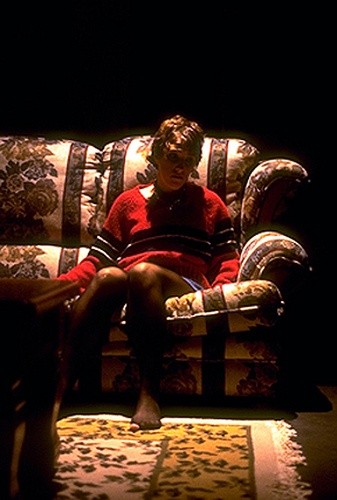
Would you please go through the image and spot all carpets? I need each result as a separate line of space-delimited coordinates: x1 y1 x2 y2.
52 412 313 500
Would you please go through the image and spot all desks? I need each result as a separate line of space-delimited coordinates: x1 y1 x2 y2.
0 278 83 499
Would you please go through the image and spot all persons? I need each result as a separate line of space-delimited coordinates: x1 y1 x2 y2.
47 112 241 430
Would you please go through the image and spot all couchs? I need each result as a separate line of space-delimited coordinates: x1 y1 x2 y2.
0 135 312 400
